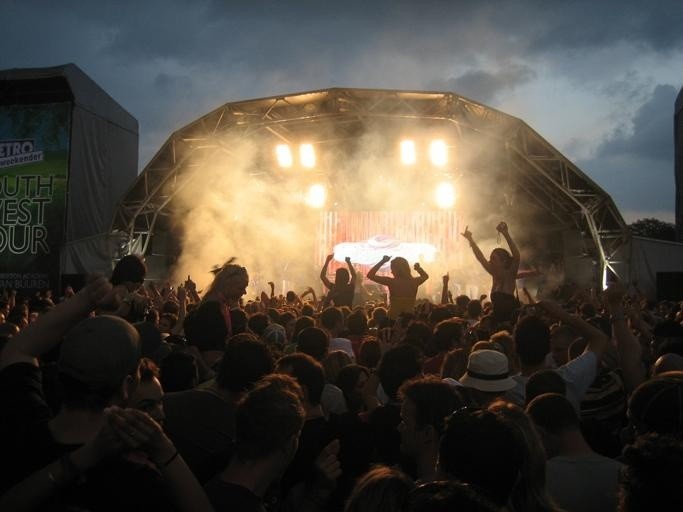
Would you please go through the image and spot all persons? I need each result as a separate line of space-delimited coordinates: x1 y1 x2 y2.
0 220 682 511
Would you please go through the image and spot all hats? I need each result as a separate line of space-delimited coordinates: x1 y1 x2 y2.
459 349 517 393
